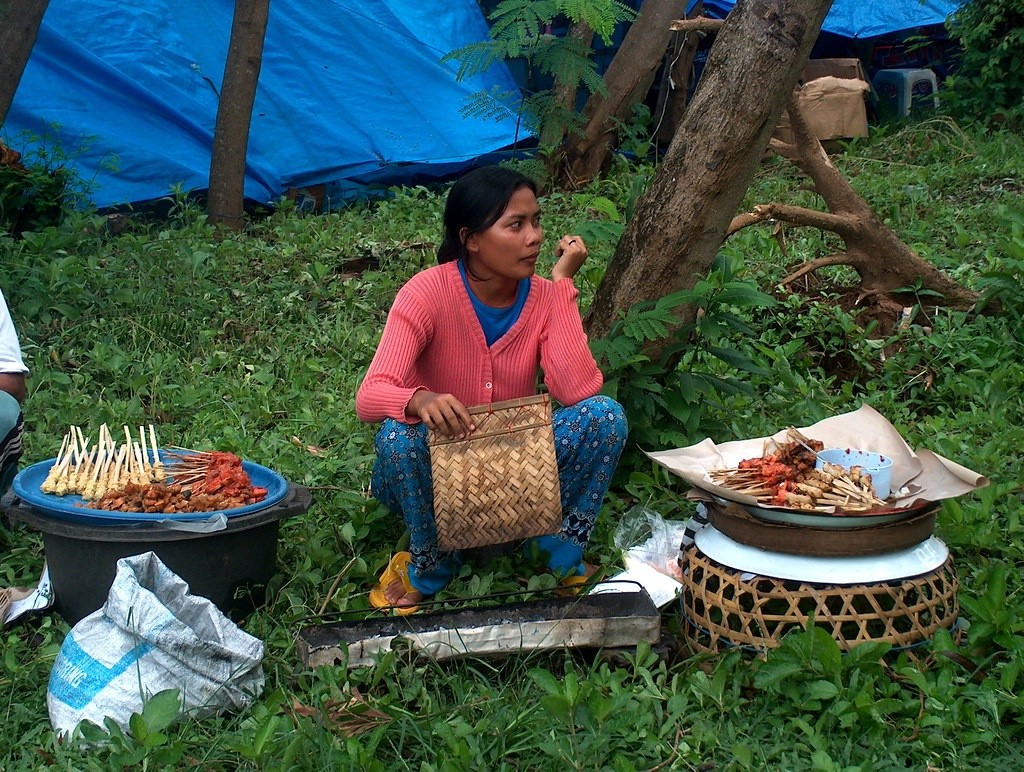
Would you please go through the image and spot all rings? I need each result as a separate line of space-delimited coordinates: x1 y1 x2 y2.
568 240 576 245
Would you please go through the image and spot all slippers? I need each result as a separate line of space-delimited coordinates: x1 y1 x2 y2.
369 551 422 616
518 562 607 596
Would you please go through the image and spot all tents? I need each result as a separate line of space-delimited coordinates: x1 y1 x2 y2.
0 0 977 216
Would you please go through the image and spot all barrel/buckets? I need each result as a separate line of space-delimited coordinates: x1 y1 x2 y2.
6 484 312 629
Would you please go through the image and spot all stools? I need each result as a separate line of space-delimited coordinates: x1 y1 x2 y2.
868 68 939 122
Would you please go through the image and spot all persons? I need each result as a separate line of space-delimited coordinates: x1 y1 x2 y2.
356 167 627 617
0 286 30 492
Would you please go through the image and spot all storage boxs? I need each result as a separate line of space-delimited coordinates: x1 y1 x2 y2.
773 58 879 155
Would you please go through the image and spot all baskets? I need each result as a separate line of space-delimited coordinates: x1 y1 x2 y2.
679 543 961 677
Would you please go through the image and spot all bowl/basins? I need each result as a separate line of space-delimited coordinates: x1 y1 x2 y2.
816 449 893 500
